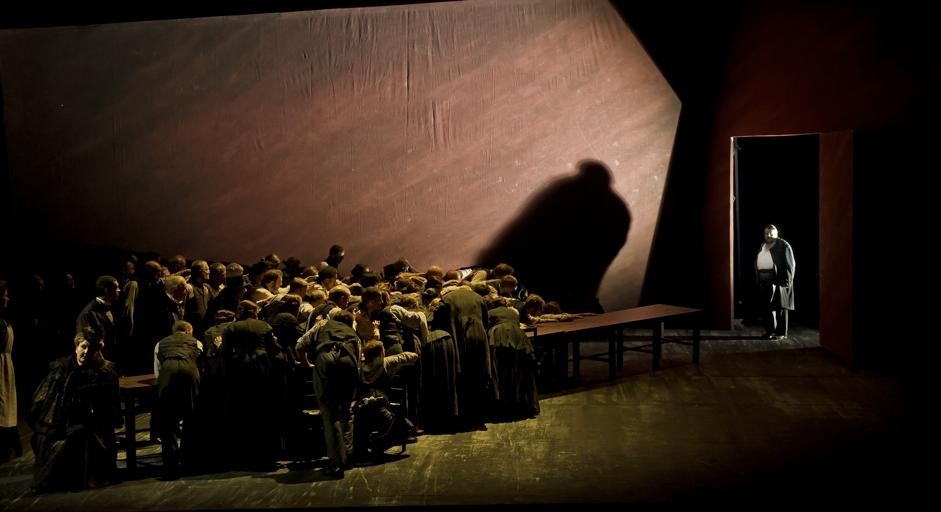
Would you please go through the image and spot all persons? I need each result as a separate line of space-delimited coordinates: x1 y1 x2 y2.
1 243 595 478
756 223 798 343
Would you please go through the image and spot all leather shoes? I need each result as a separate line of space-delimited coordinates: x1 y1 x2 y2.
763 332 787 339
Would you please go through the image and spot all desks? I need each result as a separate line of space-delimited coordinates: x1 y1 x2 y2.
118 303 705 480
